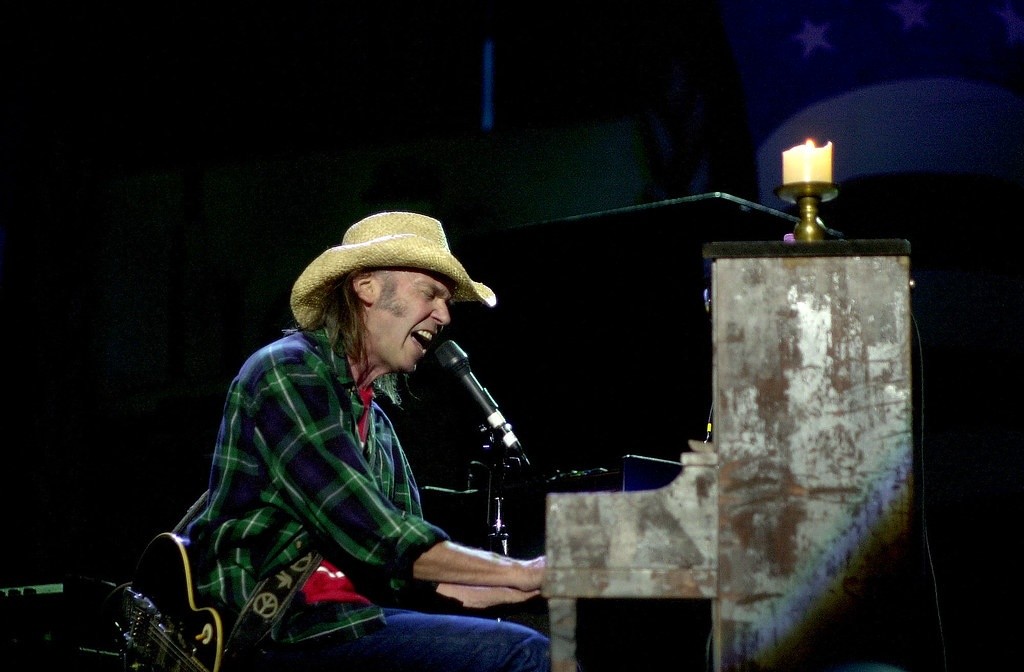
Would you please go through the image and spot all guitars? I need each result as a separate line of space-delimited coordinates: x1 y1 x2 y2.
104 527 234 672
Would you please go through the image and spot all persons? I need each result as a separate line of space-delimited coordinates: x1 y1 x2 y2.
179 212 581 672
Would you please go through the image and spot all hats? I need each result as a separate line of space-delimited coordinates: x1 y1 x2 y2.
289 211 497 331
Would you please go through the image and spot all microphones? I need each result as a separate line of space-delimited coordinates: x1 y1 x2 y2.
435 340 531 467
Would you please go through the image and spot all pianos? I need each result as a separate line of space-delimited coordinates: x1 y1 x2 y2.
544 235 926 672
